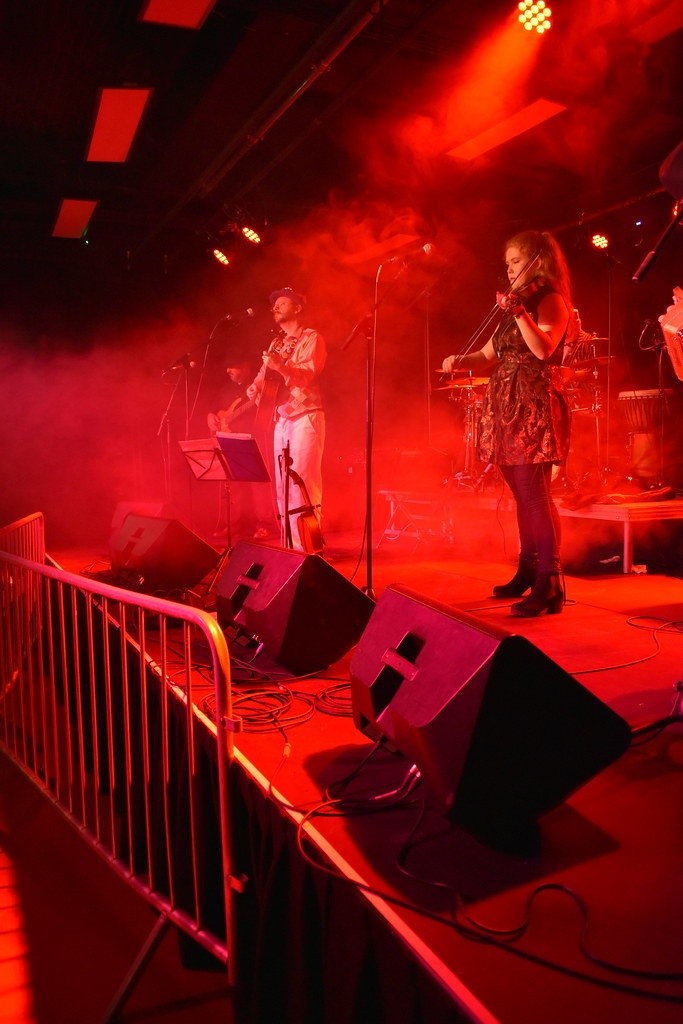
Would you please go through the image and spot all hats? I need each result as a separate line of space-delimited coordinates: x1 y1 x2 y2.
268 287 307 309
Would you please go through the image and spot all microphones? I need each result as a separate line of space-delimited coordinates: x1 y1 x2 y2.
172 361 196 370
226 308 254 320
380 243 435 266
602 252 620 264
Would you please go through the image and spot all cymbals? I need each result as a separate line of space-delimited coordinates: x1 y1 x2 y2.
446 377 490 385
435 385 478 389
585 337 608 344
435 367 468 373
567 389 581 394
574 356 616 368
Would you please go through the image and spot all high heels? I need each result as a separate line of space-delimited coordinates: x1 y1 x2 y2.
511 556 564 615
494 553 538 598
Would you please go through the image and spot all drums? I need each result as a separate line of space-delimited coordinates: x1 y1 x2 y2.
466 401 483 417
618 388 672 477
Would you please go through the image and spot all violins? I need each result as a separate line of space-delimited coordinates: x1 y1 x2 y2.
502 273 548 315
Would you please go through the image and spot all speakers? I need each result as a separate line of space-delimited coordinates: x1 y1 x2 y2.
349 583 631 836
214 540 376 675
110 500 220 597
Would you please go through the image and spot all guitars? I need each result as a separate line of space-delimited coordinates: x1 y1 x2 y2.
210 397 255 439
254 330 288 431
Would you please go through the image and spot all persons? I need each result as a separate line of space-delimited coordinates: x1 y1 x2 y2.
245 287 328 563
206 343 290 541
550 305 596 486
442 232 574 613
